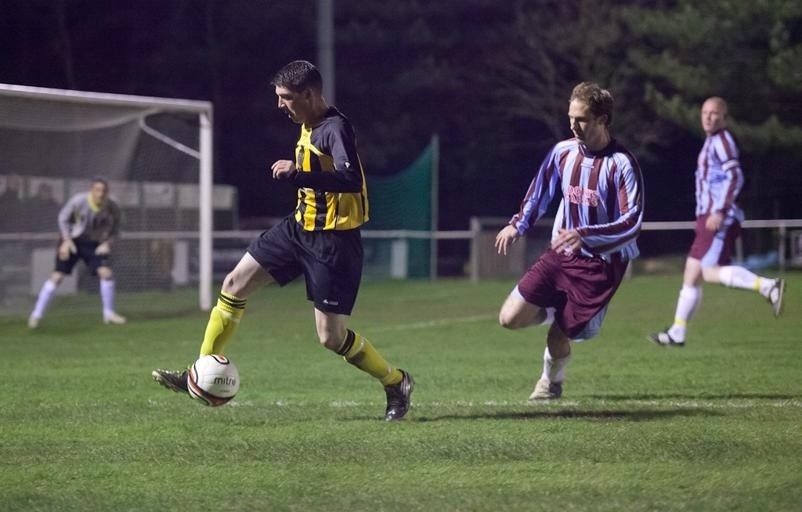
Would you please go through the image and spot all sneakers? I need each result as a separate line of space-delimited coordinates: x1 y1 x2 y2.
528 373 562 401
766 278 786 318
384 368 416 422
28 309 40 330
152 365 190 395
644 327 686 347
103 313 127 324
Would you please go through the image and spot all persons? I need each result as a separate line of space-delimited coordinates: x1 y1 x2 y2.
27 178 126 330
495 81 645 402
648 96 786 347
153 59 415 422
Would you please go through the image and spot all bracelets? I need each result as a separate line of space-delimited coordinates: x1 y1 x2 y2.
288 169 298 183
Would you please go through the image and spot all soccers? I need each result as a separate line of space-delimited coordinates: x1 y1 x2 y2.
187 354 239 408
